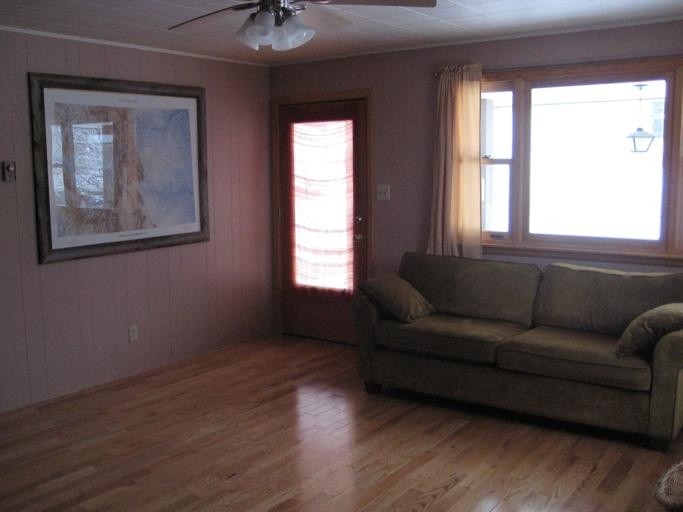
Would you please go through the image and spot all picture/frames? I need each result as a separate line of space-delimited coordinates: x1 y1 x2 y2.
27 71 209 264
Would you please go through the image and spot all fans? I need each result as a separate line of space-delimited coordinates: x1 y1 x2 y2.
167 0 437 31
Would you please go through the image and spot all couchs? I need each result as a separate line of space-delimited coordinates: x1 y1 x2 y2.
352 251 683 453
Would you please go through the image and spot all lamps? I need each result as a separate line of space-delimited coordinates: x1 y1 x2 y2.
626 126 655 152
233 11 315 52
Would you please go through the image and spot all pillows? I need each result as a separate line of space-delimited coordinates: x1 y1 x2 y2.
618 304 683 355
358 272 436 324
